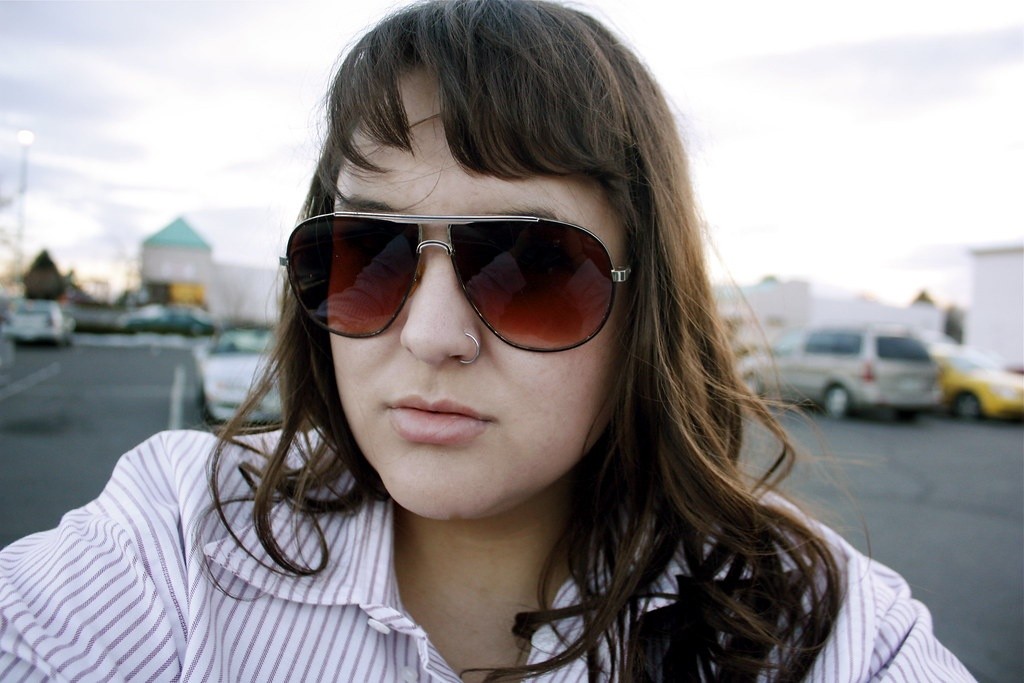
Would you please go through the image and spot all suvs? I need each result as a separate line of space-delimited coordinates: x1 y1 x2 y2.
760 320 944 423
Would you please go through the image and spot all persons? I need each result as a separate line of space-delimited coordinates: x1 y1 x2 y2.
0 1 976 683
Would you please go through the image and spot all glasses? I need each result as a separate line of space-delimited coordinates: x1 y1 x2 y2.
281 210 629 354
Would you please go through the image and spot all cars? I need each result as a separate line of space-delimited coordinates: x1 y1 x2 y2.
902 337 1024 428
10 296 77 345
112 300 211 336
196 328 288 428
